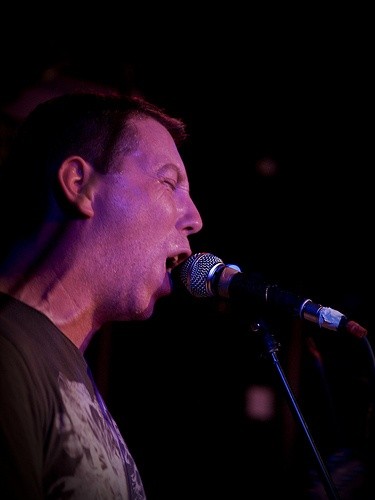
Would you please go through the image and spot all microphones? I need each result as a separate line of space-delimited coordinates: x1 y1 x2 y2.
180 251 367 339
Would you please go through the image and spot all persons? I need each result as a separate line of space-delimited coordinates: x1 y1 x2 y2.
0 83 202 500
